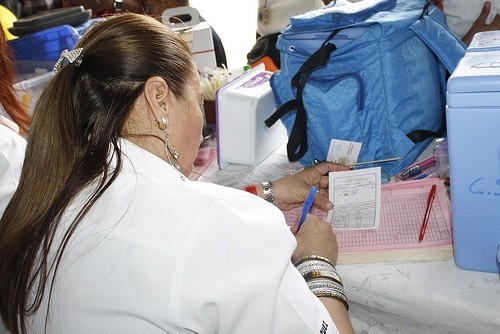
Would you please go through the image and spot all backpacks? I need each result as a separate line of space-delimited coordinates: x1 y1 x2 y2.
276 0 465 176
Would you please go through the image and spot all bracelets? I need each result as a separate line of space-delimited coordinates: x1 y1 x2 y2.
260 180 279 209
242 184 258 197
290 256 351 310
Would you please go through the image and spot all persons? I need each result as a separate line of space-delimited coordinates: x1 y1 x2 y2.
0 14 354 334
0 19 31 222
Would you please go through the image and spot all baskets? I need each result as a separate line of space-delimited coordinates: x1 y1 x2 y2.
203 100 216 136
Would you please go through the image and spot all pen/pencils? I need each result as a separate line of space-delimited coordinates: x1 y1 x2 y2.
294 185 319 236
401 154 436 180
419 184 437 241
